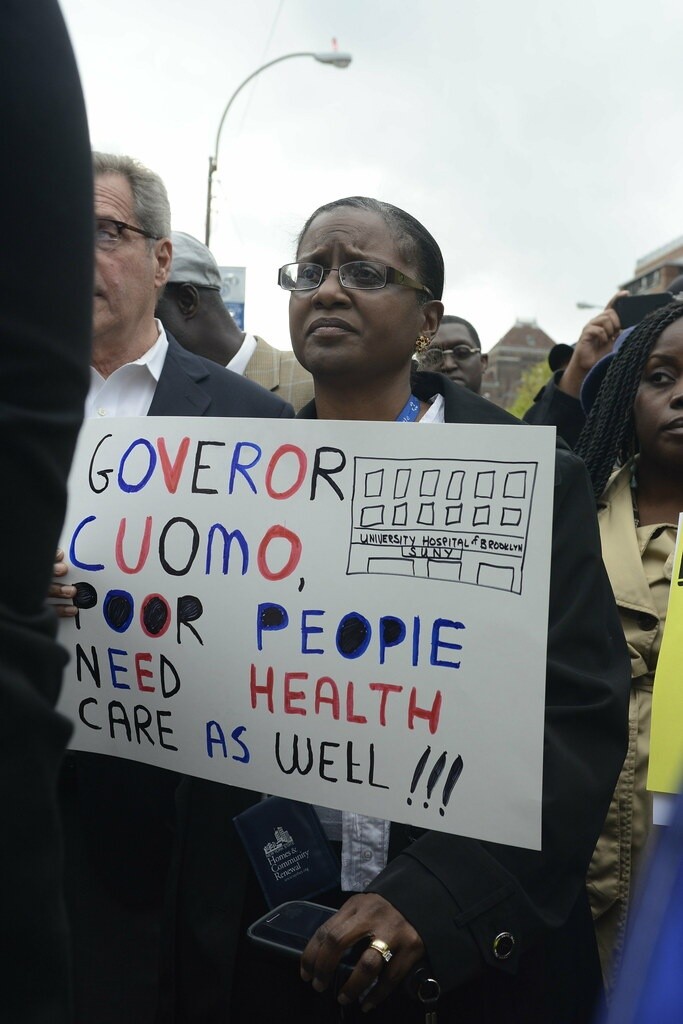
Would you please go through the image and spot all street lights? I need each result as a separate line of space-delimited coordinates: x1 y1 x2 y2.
201 50 357 250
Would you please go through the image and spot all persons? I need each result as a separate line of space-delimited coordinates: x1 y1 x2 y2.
0 0 682 1024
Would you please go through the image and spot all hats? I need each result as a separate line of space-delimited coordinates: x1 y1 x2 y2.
167 231 223 288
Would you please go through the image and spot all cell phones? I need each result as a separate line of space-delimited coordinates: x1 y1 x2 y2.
246 901 373 973
614 292 673 330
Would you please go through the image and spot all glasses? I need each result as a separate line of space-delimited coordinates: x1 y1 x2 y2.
419 345 481 368
95 217 162 254
279 260 433 297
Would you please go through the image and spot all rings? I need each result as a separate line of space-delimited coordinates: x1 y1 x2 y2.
368 937 394 964
